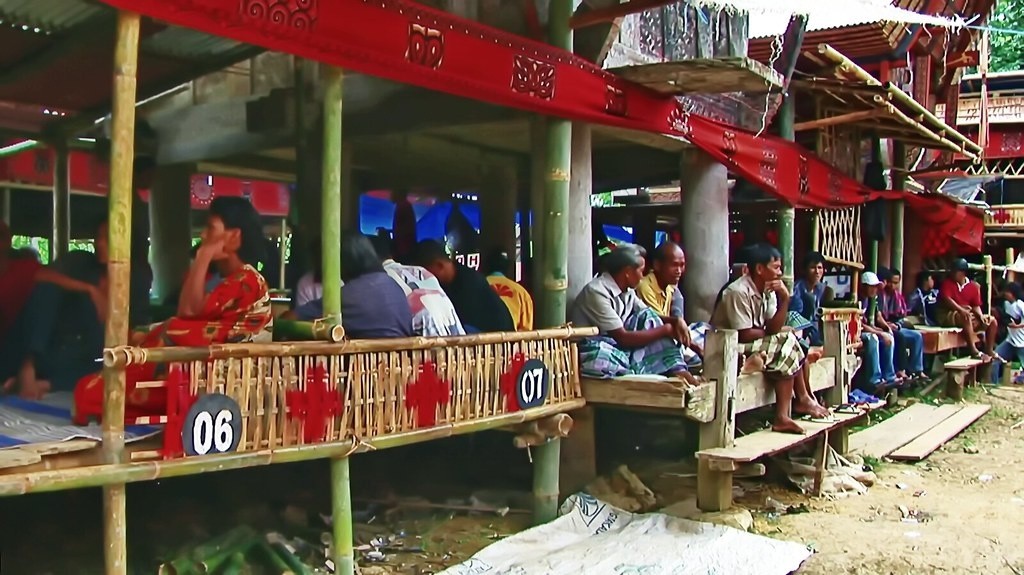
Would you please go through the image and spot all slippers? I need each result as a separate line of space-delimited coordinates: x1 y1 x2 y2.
833 401 871 414
990 352 999 359
801 414 841 423
874 375 933 388
971 351 985 360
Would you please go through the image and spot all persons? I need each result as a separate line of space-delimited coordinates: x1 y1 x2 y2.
0 155 1023 443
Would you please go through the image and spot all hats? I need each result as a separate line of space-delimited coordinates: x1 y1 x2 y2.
861 271 884 286
